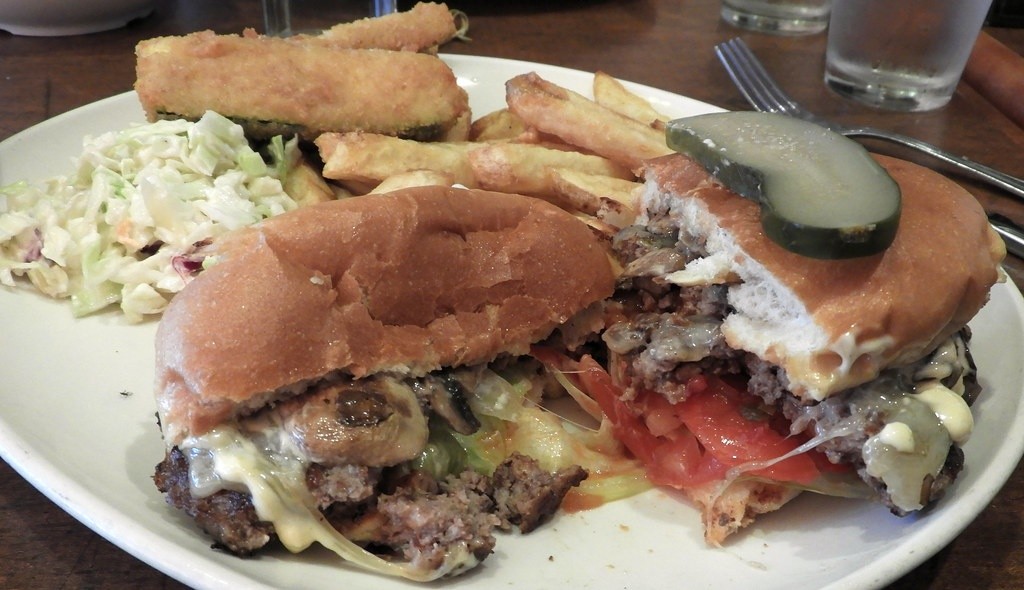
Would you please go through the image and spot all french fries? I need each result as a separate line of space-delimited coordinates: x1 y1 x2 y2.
312 69 676 236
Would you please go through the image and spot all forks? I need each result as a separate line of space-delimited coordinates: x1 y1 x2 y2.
711 35 1024 206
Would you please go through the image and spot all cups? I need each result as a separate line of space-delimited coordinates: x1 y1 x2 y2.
822 0 995 113
719 0 831 37
258 1 399 42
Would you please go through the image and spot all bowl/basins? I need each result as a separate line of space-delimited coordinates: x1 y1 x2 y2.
0 0 153 37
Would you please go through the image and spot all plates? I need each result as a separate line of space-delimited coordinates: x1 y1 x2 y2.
0 53 1024 590
